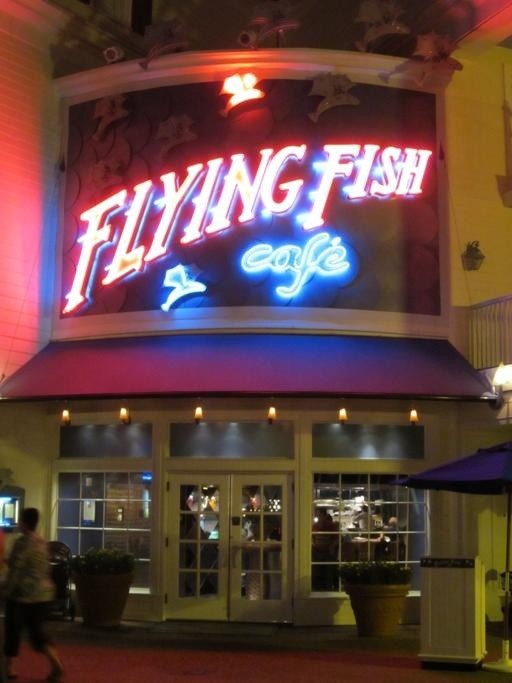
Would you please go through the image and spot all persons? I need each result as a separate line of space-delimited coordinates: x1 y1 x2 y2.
313 500 405 590
0 506 66 682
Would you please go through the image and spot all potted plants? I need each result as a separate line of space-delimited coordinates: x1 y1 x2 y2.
336 559 417 637
66 545 136 628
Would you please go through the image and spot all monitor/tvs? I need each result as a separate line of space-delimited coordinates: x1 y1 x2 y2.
0 497 19 525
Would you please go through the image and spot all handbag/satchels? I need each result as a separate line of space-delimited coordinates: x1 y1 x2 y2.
52 563 68 598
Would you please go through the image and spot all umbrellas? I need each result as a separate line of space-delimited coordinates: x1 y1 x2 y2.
392 439 512 661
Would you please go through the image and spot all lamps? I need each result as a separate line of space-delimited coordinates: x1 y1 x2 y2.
194 407 204 425
463 240 485 271
409 408 419 425
266 407 277 426
61 410 73 428
102 44 126 64
338 408 348 426
120 407 131 425
236 29 257 49
